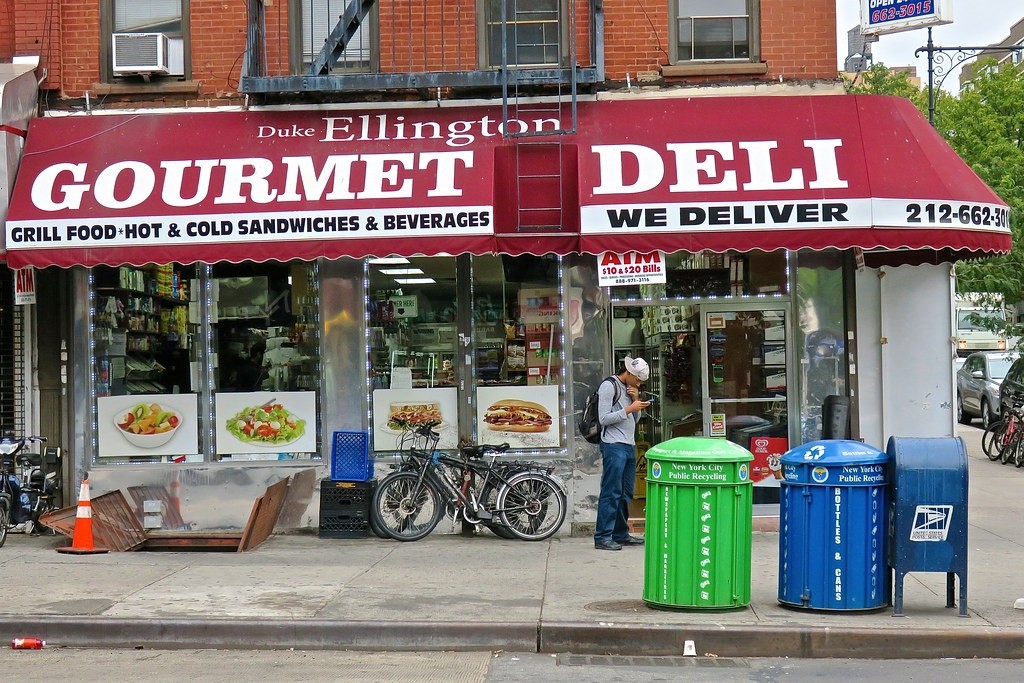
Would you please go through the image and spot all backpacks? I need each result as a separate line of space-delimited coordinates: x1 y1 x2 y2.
579 377 622 444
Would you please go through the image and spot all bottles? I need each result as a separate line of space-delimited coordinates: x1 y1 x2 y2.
296 375 314 386
384 333 397 348
371 370 390 389
378 300 394 320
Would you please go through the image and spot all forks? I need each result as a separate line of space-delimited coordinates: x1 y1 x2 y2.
243 398 276 421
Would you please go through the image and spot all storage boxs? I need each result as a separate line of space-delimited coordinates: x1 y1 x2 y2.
319 429 379 539
640 304 689 345
517 288 560 386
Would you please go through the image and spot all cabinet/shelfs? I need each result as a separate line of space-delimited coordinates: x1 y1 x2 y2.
761 311 787 396
94 286 189 393
219 316 319 391
370 326 408 389
504 320 526 382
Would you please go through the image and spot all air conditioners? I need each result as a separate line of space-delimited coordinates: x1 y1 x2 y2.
112 32 170 75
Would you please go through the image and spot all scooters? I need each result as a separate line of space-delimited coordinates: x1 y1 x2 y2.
0 430 65 548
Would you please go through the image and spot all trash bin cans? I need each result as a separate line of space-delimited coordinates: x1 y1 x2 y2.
726 415 768 436
777 439 889 614
890 435 969 619
643 436 755 613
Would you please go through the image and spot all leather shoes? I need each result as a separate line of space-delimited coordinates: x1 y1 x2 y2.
595 539 622 550
615 535 645 546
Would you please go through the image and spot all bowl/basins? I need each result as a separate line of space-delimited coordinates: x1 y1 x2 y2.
114 404 183 448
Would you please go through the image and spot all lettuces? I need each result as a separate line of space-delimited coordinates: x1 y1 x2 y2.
226 406 305 444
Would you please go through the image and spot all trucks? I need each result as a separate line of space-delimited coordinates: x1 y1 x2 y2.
954 306 1007 359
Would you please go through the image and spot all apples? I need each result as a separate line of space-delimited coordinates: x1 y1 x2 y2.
117 413 134 428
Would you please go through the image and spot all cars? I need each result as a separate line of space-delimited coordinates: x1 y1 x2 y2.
998 354 1024 426
956 349 1022 431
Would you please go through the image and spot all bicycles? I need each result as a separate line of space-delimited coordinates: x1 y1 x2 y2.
982 392 1024 470
367 415 567 542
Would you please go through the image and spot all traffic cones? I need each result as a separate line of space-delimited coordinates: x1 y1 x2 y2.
54 479 111 556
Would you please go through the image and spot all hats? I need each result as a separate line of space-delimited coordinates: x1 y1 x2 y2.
625 356 650 381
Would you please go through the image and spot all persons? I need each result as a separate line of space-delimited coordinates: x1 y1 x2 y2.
594 356 651 550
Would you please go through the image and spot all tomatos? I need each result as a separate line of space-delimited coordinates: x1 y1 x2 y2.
243 404 293 437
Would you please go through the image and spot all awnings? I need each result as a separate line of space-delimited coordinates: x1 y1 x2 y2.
3 95 1012 271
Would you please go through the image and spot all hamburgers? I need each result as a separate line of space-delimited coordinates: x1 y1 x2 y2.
387 402 442 430
482 400 552 432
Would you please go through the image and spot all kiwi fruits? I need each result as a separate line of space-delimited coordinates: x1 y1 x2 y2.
132 404 149 421
154 412 169 426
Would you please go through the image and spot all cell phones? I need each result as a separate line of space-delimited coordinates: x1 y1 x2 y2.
648 399 654 402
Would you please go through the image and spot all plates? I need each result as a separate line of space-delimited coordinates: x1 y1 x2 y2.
381 420 450 436
228 408 304 446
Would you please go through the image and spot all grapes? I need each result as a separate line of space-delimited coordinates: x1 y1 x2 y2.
169 416 180 428
